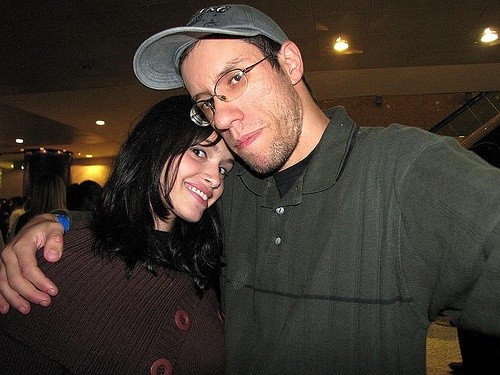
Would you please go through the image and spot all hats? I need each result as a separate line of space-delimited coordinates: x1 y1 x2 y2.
133 4 290 90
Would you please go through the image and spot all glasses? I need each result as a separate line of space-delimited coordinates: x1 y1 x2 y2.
190 53 272 127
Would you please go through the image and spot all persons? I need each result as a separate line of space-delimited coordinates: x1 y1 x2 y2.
0 93 235 375
0 4 500 375
0 172 102 252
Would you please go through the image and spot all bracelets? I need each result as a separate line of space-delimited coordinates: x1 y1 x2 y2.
56 213 70 233
